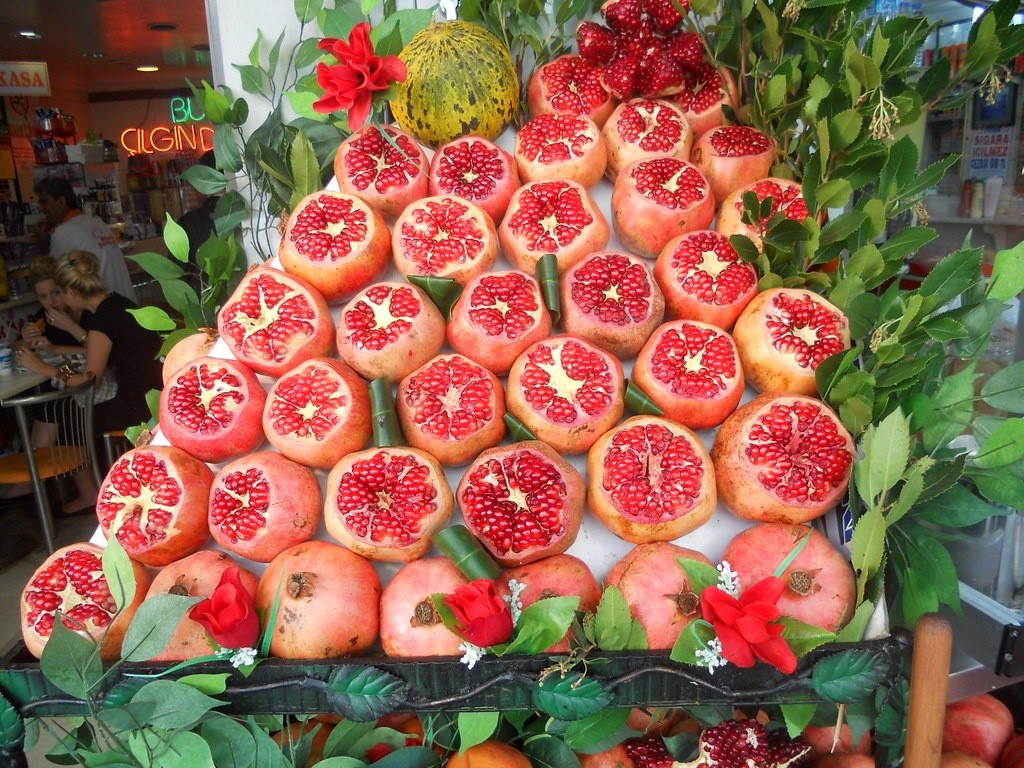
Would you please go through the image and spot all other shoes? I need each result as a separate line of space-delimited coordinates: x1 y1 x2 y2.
50 500 98 518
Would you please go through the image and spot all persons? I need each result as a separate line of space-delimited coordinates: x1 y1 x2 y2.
33 175 138 303
168 149 226 304
15 251 163 518
12 255 93 395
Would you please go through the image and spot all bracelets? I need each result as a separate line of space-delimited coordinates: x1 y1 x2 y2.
81 333 88 345
51 365 72 391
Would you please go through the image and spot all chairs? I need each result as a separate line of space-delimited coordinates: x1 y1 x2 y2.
0 371 103 559
102 356 167 469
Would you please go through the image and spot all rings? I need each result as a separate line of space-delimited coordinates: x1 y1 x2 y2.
50 318 54 322
16 349 21 354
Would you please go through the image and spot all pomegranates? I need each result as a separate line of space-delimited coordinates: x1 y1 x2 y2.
21 0 1023 768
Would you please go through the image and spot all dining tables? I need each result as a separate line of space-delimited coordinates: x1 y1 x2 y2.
0 352 74 400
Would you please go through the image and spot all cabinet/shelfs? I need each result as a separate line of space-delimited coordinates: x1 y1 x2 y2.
899 216 1024 294
0 160 204 341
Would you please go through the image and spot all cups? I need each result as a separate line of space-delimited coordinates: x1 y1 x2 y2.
0 338 11 348
0 349 13 375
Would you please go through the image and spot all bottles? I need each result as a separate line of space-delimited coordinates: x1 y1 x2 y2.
8 277 20 301
29 197 37 215
